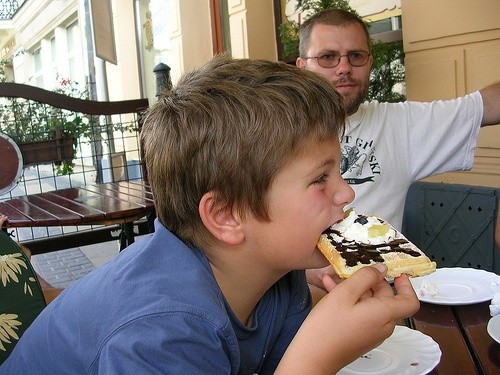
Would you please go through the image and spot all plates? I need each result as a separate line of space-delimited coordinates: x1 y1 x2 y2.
409 267 500 306
335 325 443 375
486 314 500 344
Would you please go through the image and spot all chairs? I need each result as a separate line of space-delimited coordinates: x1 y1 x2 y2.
0 215 47 366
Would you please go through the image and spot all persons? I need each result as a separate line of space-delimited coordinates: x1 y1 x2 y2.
0 46 420 375
295 8 500 234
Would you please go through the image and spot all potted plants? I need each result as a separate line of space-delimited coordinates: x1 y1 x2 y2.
0 60 140 177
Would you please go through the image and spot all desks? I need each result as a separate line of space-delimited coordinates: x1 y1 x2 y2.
0 180 156 253
393 298 500 375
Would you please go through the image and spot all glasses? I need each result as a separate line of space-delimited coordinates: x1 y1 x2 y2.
305 51 371 68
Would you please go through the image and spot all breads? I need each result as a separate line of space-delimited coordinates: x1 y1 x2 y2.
316 212 437 279
